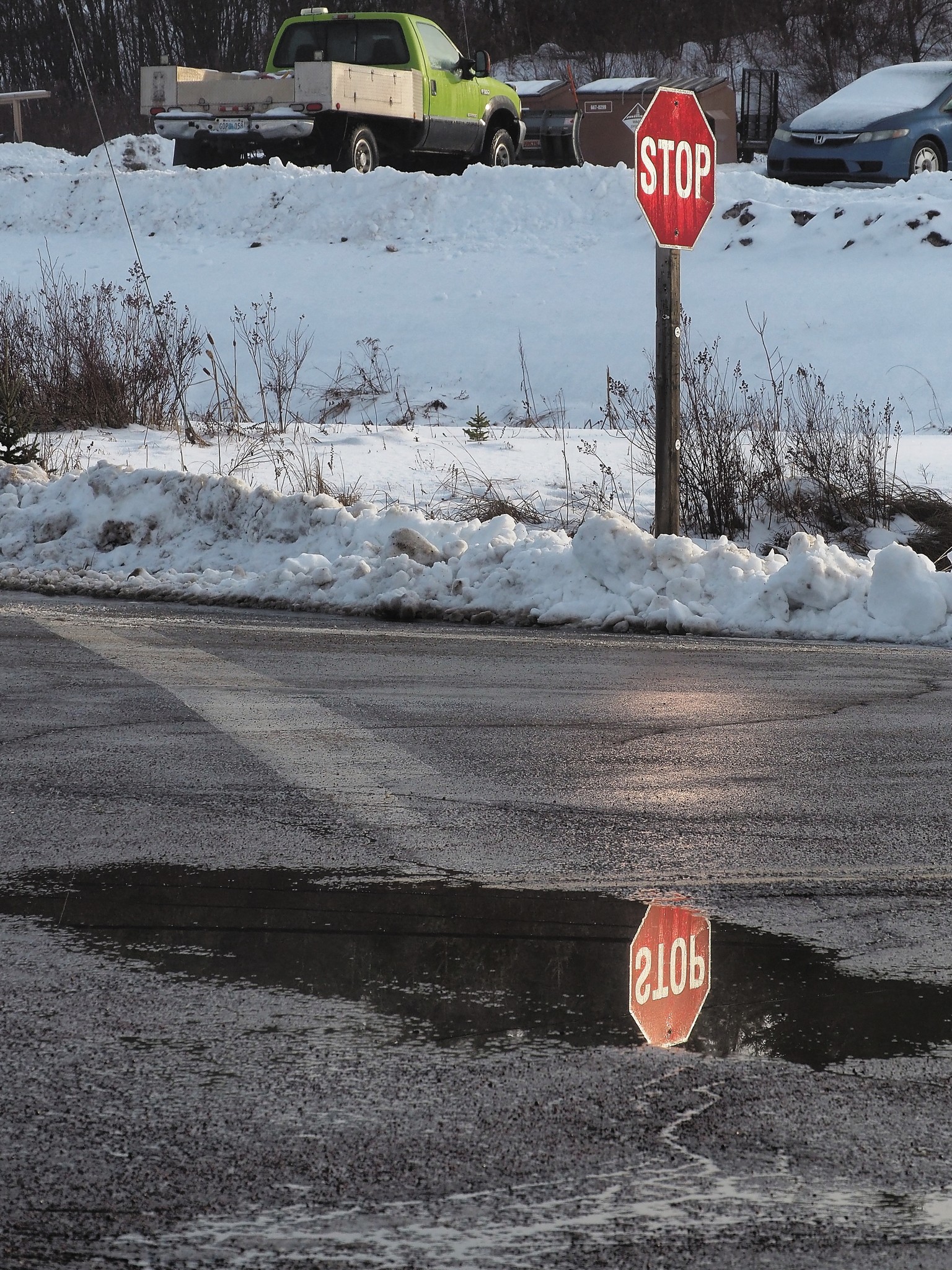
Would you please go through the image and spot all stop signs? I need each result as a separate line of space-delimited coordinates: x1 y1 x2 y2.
635 84 716 249
627 894 714 1047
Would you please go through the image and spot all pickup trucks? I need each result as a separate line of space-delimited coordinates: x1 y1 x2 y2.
139 5 528 173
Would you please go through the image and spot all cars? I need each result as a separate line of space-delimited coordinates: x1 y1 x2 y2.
767 60 952 184
